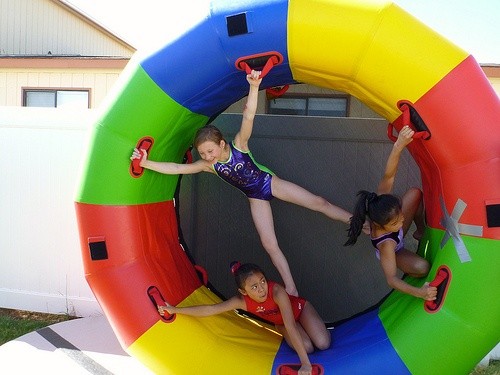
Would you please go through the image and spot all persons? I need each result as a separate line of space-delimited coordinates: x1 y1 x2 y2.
130 69 373 297
156 263 334 375
342 125 438 302
